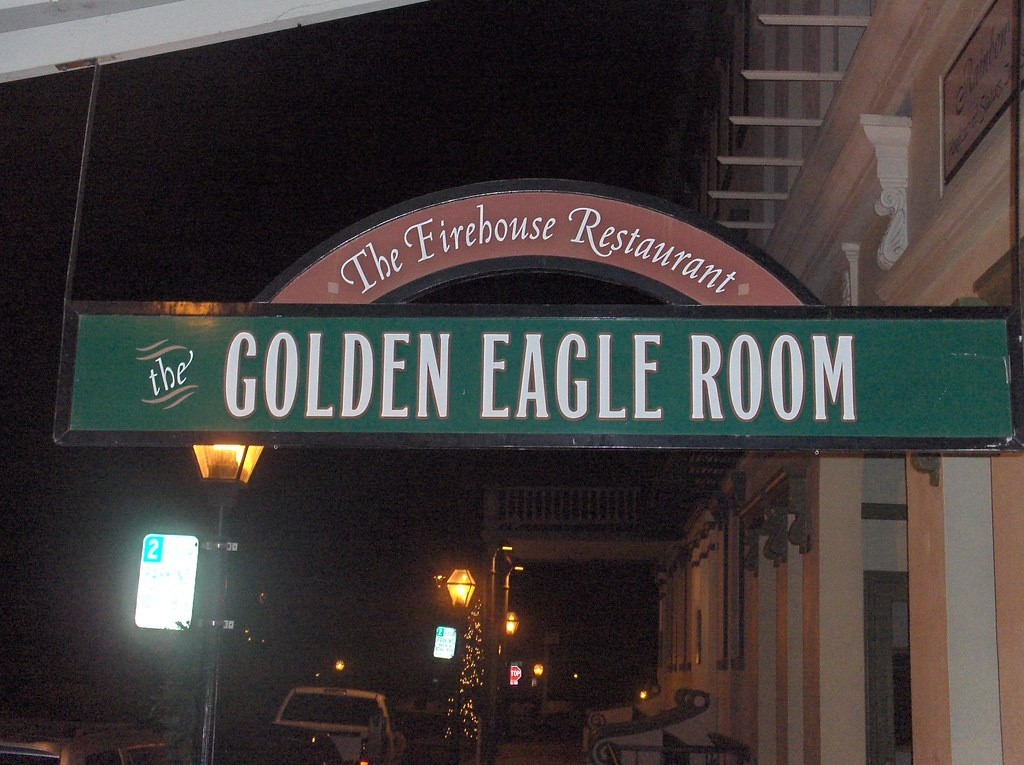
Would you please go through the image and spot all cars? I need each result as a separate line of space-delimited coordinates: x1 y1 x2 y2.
0 715 194 765
270 683 398 765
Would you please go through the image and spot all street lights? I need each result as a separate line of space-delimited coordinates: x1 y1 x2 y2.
445 567 477 765
189 444 267 765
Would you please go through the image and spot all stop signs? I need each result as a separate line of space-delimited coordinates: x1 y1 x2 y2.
511 665 522 681
